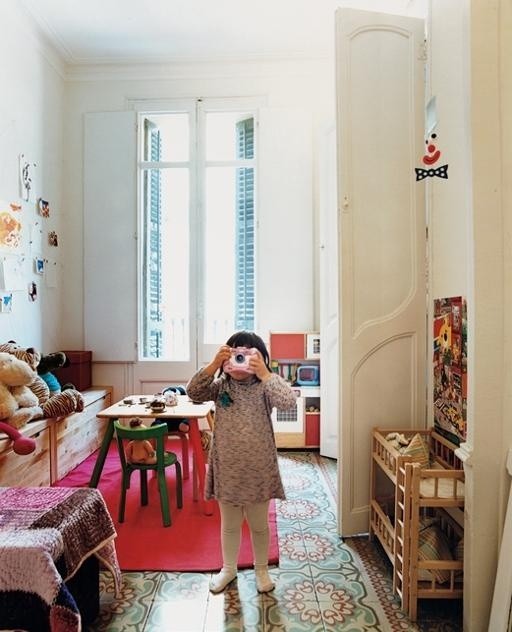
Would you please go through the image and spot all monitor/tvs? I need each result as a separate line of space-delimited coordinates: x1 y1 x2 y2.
297 366 320 386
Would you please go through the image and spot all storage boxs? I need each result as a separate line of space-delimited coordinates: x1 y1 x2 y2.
54 351 92 391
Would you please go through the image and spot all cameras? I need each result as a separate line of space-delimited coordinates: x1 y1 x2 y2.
223 347 258 375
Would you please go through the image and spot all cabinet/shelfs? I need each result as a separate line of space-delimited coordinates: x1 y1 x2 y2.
270 330 320 449
368 426 465 622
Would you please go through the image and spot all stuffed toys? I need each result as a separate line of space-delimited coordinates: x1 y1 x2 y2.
123 417 158 466
149 384 189 433
0 339 83 455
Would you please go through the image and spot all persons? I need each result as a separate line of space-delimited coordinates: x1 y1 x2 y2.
186 330 299 593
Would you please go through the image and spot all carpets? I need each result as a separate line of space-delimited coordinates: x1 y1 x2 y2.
52 439 279 572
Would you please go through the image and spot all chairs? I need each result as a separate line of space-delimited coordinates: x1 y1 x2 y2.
114 422 182 527
152 386 190 481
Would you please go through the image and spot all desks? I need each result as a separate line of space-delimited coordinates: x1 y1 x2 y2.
89 394 214 516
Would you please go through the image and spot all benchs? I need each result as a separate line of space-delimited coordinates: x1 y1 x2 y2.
0 386 112 487
